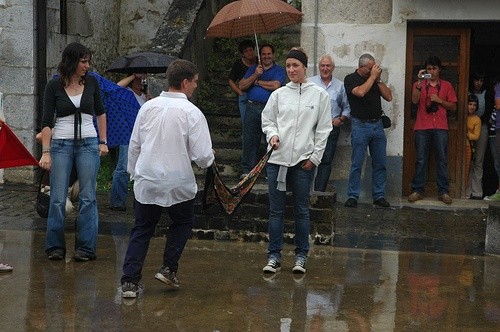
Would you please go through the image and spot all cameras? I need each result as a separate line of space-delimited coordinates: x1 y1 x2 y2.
420 72 432 80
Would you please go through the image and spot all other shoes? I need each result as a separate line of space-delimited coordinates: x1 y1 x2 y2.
0 262 13 272
408 191 424 201
473 196 480 200
107 206 127 214
372 197 390 208
48 251 64 261
345 198 357 207
73 252 97 261
313 195 332 209
438 193 453 204
484 189 500 202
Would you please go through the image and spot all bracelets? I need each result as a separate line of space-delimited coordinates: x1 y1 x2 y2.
416 85 422 90
338 117 344 122
256 79 259 86
98 141 107 144
42 148 51 152
375 79 383 83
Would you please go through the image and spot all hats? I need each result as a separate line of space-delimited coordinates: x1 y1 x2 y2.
238 39 255 54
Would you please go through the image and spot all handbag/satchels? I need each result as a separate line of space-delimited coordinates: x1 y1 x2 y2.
381 115 392 129
37 165 50 219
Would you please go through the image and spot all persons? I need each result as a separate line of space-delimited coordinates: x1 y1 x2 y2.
34 40 153 261
120 59 216 299
464 72 500 202
261 47 334 273
344 54 392 207
0 115 14 273
305 54 352 192
229 41 286 180
408 56 457 204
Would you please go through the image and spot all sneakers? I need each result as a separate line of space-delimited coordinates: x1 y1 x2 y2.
292 254 307 273
154 265 180 288
263 255 282 273
120 280 145 298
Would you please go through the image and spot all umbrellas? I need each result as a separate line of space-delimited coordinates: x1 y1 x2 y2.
52 71 141 148
204 0 304 65
105 51 181 73
